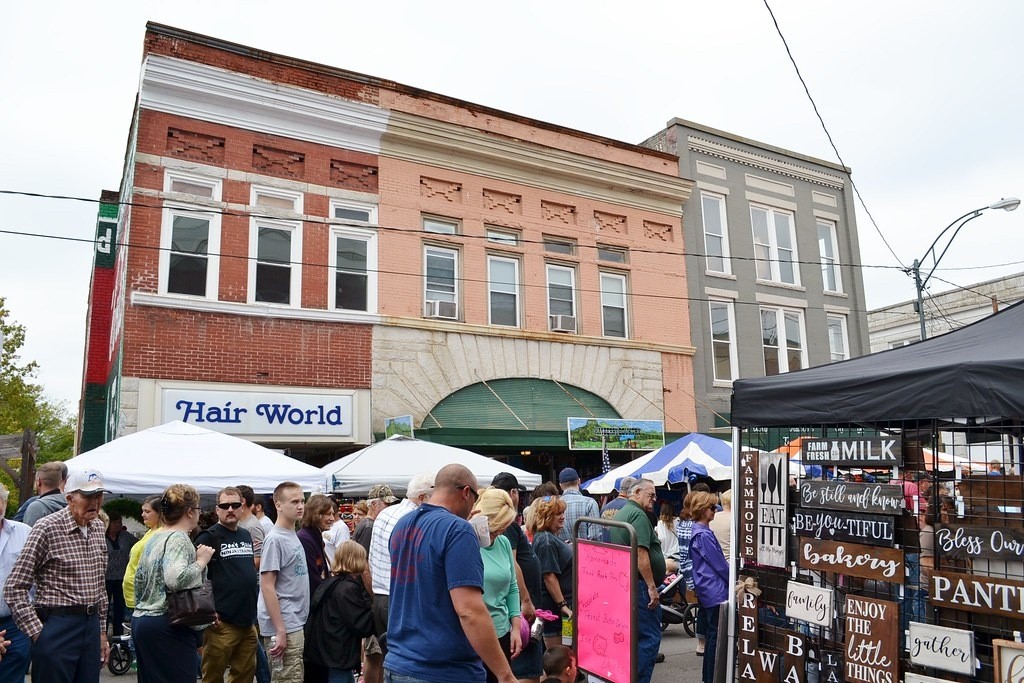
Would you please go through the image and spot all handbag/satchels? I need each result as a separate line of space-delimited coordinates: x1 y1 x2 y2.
165 581 217 628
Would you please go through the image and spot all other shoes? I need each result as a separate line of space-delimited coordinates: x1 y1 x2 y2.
656 653 665 663
696 646 705 655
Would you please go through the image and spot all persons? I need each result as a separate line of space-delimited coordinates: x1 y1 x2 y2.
754 459 1014 683
0 460 732 683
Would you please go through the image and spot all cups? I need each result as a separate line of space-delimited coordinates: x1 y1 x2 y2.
469 516 491 548
562 617 573 645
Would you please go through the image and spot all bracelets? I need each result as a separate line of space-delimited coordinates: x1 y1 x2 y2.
558 600 567 610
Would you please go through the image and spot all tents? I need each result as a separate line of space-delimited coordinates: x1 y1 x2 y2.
321 434 541 499
63 420 326 509
771 438 987 473
727 298 1024 683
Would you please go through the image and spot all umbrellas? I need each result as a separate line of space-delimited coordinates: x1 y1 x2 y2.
580 433 820 495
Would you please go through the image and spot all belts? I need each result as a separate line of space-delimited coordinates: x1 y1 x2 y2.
47 603 99 615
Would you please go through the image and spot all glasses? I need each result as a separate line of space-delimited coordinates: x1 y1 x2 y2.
218 502 243 510
708 506 718 511
640 489 656 498
191 507 204 512
430 485 479 502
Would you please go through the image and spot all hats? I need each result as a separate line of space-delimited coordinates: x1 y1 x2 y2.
914 471 931 480
65 469 114 496
559 468 578 484
367 484 400 503
491 472 527 491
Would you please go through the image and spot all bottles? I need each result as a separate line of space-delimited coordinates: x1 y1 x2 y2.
270 636 283 672
132 657 137 672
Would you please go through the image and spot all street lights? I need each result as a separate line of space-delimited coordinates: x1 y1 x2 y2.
913 197 1020 338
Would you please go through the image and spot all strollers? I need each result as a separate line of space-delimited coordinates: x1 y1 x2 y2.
107 620 134 675
655 573 700 638
